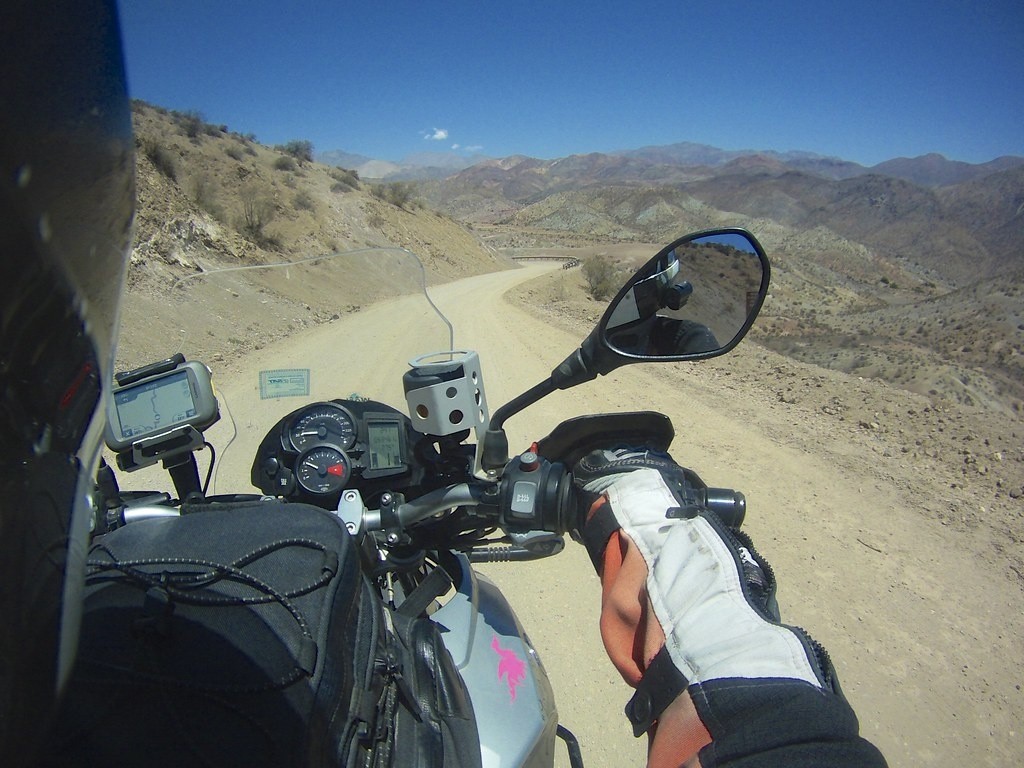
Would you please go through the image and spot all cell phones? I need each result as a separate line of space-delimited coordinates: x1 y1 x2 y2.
103 361 218 453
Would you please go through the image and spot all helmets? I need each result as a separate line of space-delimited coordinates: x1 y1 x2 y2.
606 249 679 335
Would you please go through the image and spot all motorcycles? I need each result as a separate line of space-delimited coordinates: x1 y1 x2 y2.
93 222 771 767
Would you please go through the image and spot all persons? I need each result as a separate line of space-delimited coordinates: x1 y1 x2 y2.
604 246 721 356
0 0 889 768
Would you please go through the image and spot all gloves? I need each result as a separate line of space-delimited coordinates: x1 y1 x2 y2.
571 441 689 533
